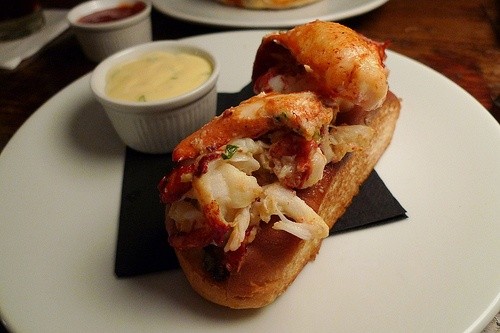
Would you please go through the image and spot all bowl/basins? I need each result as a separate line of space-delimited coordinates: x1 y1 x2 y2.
90 40 220 152
66 0 152 61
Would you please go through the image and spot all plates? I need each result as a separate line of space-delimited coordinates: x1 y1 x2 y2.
1 9 72 70
152 1 387 28
1 29 500 333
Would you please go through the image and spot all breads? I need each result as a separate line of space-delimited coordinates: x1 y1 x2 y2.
168 91 402 310
